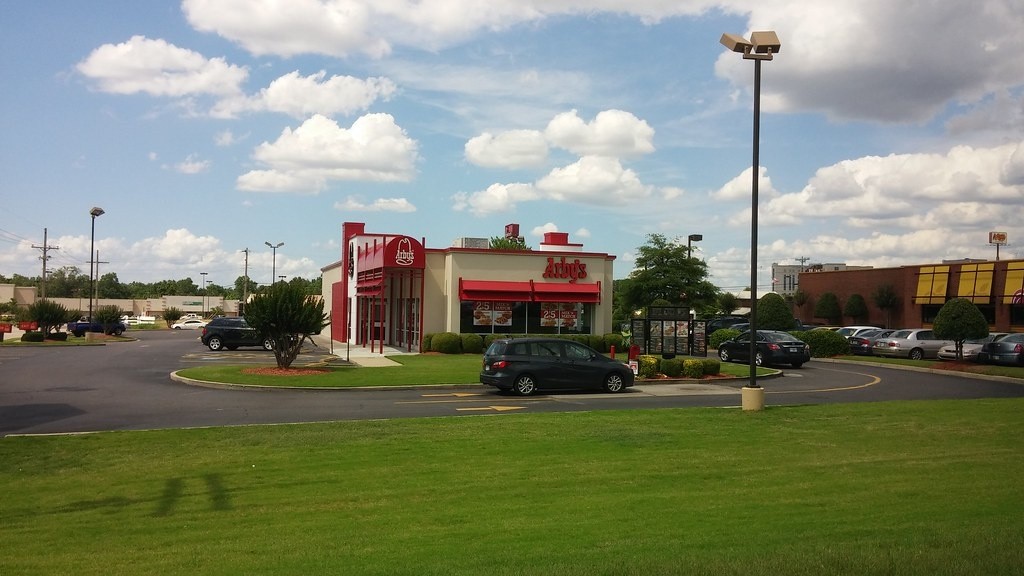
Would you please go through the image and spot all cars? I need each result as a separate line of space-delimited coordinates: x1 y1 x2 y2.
201 317 298 351
707 317 1024 368
171 320 208 330
718 330 810 367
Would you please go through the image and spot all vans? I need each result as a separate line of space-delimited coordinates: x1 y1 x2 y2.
480 338 634 396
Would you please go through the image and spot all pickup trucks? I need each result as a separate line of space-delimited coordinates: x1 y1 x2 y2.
67 319 126 337
180 314 202 319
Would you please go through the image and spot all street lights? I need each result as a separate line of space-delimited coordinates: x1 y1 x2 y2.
90 206 105 324
265 242 284 285
687 234 702 321
